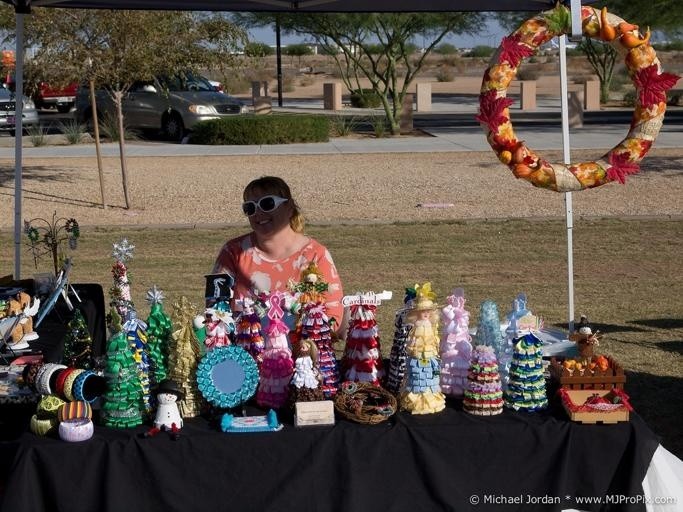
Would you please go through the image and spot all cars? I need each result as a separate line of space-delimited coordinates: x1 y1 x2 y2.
0 82 38 138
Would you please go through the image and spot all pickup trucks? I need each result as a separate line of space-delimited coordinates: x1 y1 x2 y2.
0 65 80 114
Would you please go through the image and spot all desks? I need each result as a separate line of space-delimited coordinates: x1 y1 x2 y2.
1 360 683 512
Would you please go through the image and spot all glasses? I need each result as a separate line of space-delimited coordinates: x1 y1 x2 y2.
242 195 288 217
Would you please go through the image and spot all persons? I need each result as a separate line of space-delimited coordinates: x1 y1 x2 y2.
0 291 40 350
212 176 344 338
97 261 550 441
570 315 600 360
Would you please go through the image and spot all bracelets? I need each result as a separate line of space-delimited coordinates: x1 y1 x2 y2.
27 360 100 442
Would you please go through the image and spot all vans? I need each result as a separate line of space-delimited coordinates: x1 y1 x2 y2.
71 64 251 143
